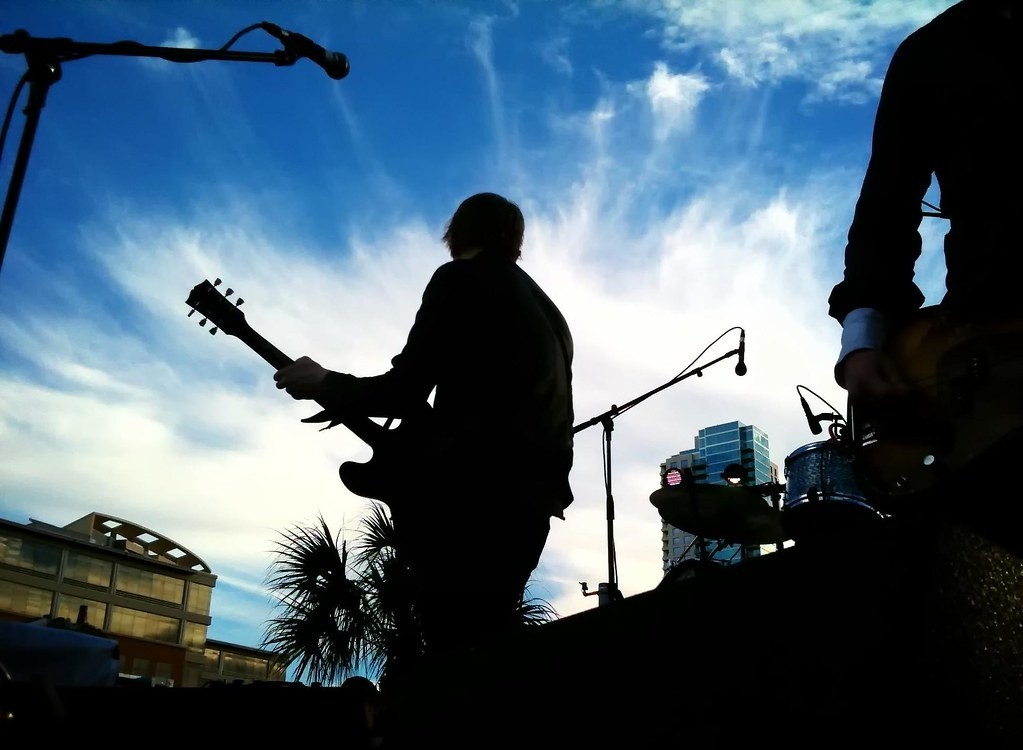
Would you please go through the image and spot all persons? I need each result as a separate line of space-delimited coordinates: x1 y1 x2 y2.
273 193 573 660
827 0 1023 471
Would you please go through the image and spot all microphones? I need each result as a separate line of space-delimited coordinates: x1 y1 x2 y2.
735 332 747 376
800 397 822 435
263 20 350 80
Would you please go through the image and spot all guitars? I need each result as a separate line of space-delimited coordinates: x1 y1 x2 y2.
185 278 408 540
854 298 1023 495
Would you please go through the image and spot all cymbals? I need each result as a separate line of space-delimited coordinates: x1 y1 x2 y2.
648 482 786 545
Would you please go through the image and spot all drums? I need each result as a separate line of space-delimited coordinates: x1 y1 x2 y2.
782 441 886 534
